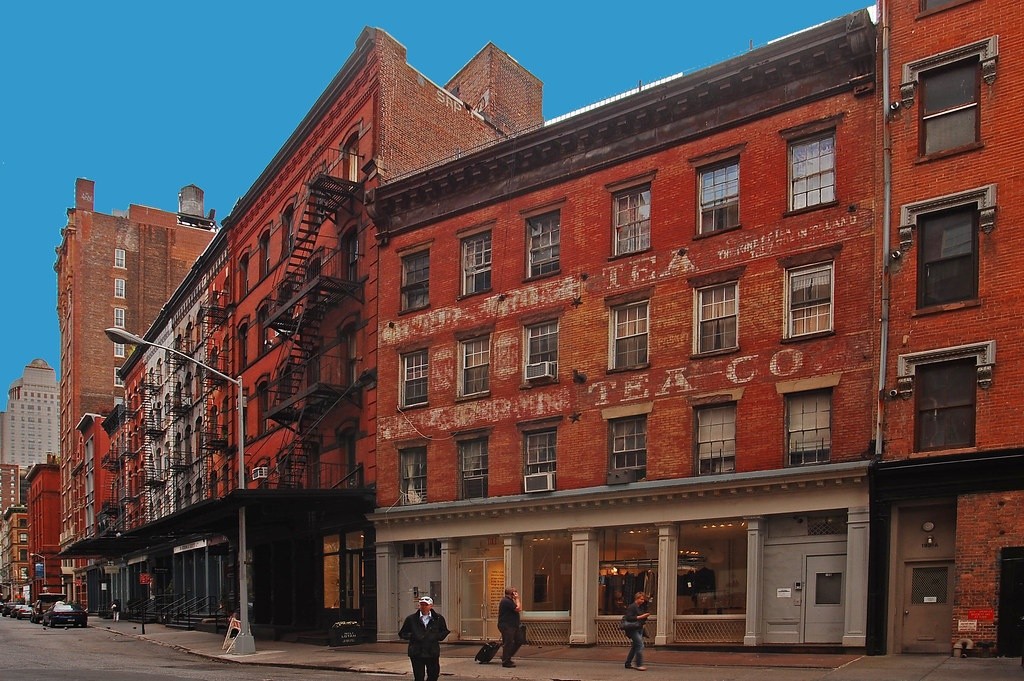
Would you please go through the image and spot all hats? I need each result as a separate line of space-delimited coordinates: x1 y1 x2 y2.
418 596 433 605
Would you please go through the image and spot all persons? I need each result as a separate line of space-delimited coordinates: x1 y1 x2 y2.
496 585 523 667
397 596 451 681
620 592 651 671
110 599 121 622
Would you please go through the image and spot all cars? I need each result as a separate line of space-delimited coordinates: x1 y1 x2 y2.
42 600 87 628
9 605 22 618
2 602 20 617
28 598 57 623
16 605 33 620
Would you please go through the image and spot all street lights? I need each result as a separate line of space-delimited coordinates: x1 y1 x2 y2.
101 323 249 632
29 552 46 585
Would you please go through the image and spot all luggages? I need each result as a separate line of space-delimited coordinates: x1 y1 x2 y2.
475 635 503 664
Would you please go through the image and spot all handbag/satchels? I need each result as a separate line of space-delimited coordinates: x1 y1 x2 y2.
618 616 643 630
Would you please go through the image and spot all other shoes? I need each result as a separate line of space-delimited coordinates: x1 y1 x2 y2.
624 665 634 668
633 665 647 670
503 663 516 667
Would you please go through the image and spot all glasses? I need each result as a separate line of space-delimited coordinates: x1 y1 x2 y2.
421 599 431 601
